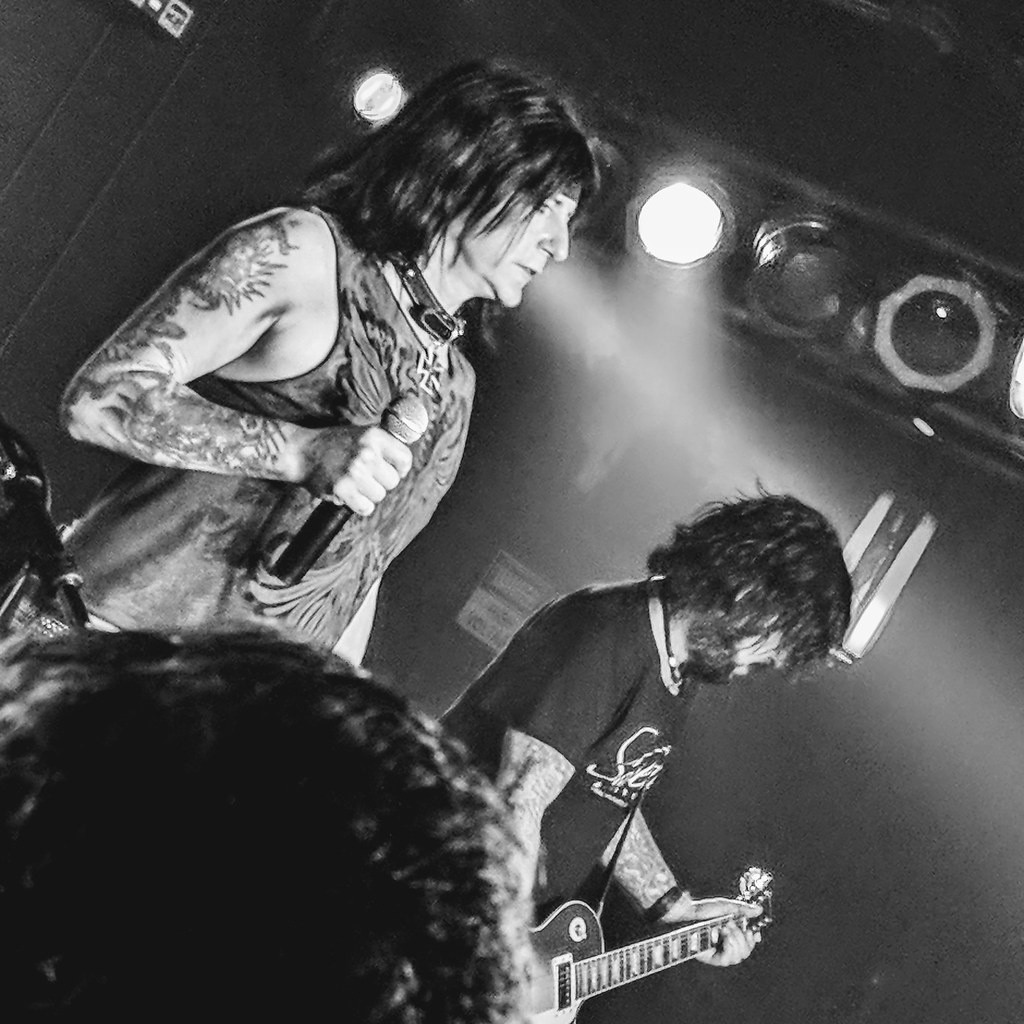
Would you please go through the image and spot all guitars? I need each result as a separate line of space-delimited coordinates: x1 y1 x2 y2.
528 864 776 1024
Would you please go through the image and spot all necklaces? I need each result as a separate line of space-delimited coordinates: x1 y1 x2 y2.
395 260 468 349
659 575 683 692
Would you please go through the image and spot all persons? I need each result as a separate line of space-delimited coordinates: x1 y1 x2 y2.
0 619 536 1024
433 494 852 1007
55 59 602 670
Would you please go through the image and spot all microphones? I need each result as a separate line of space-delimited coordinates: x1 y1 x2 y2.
268 397 429 588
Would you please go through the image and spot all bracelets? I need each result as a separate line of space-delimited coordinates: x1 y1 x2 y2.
642 882 687 924
